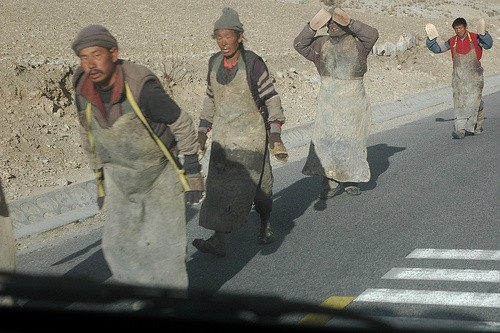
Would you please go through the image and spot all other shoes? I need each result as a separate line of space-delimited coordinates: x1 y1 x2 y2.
345 186 361 195
452 130 464 139
319 186 341 200
192 232 232 256
258 219 274 244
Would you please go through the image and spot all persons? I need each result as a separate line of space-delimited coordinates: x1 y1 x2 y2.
70 24 203 311
193 8 289 258
425 18 493 138
292 6 379 201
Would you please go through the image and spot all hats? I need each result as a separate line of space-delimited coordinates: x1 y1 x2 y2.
72 23 117 56
213 6 244 32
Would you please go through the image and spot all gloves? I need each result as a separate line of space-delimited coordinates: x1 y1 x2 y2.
183 155 204 204
192 132 208 151
268 132 289 162
